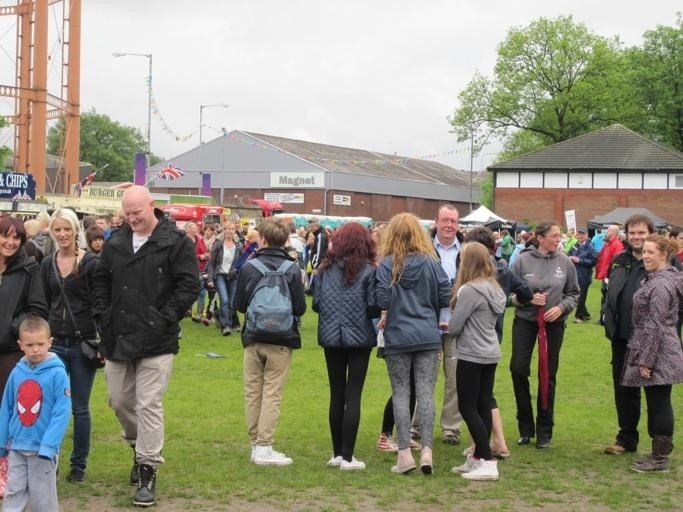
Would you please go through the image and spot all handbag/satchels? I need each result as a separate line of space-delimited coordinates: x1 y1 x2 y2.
80 337 108 370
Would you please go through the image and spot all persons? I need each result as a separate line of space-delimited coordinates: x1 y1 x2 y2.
512 217 581 453
372 210 452 477
369 224 681 355
455 225 534 462
601 211 681 458
404 201 467 445
618 232 682 477
309 221 379 473
0 315 73 511
91 184 203 507
375 316 428 456
0 215 49 406
4 210 125 266
233 215 306 469
177 214 341 338
443 239 510 483
38 208 104 484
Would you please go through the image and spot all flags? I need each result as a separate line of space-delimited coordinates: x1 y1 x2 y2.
72 169 100 199
154 164 186 183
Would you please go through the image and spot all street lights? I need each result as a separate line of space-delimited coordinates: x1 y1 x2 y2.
111 50 156 189
199 104 232 145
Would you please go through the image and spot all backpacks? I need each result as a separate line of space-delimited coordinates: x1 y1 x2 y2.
244 257 296 338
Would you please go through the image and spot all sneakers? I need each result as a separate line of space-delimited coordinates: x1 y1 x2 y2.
128 453 157 508
185 308 243 336
66 466 87 483
574 313 592 324
326 454 368 471
250 444 294 466
452 455 501 483
604 443 629 456
375 427 556 459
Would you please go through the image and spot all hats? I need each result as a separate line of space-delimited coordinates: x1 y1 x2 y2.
577 226 589 233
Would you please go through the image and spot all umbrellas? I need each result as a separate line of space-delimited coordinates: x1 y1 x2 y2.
534 289 549 419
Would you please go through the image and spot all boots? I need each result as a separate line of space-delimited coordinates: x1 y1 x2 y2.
630 435 675 473
390 448 418 475
418 447 437 475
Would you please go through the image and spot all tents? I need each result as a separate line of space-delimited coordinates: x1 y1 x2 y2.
586 207 673 246
459 206 518 243
